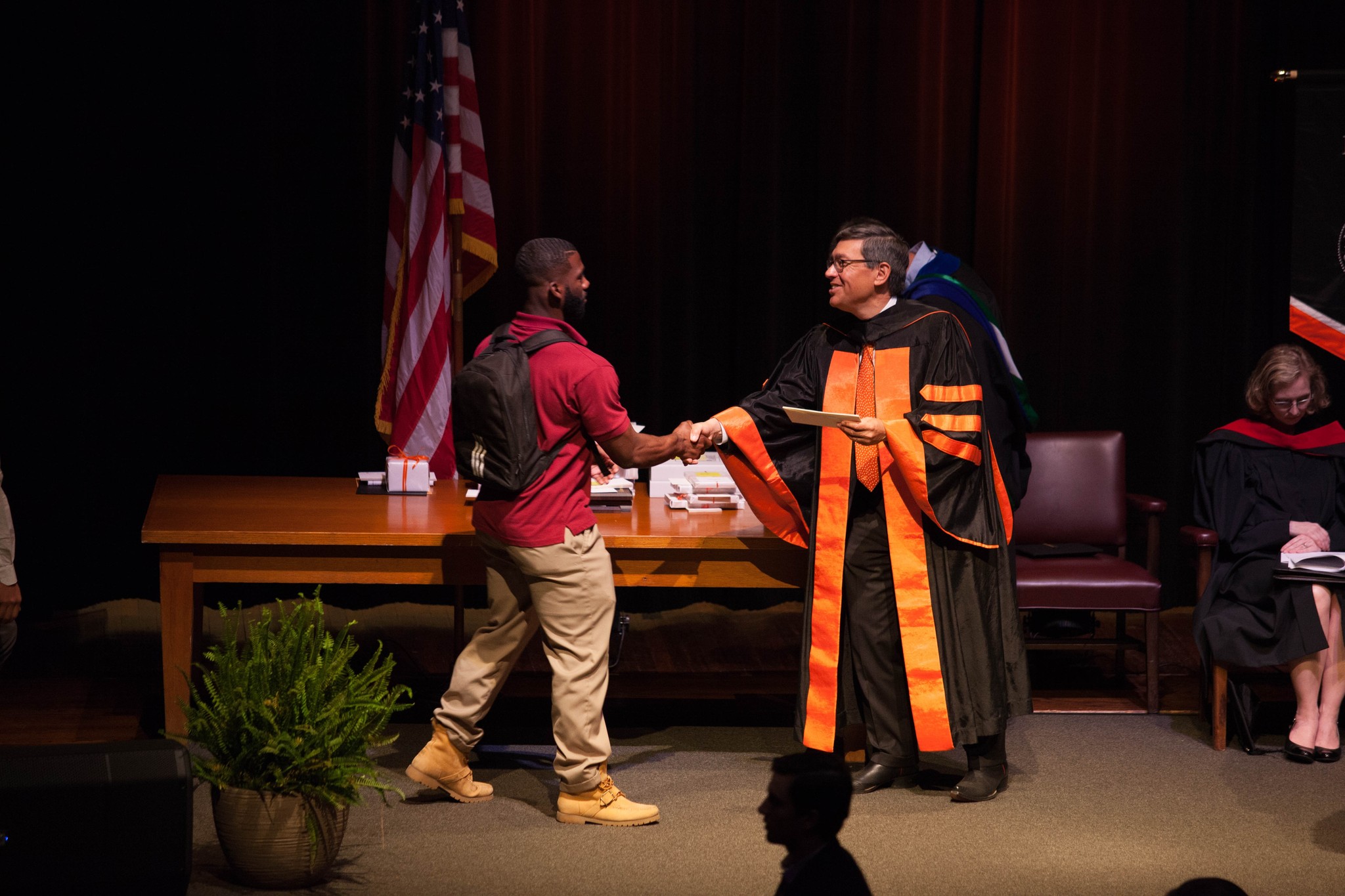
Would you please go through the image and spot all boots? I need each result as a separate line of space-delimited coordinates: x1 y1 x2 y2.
405 719 494 803
556 775 660 827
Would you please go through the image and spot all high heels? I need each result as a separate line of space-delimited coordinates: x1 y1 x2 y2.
1313 744 1341 763
1284 716 1314 761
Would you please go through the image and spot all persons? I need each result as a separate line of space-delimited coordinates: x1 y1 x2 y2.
758 751 873 896
0 470 22 627
900 241 1033 536
1201 346 1345 764
406 239 710 826
681 223 1033 803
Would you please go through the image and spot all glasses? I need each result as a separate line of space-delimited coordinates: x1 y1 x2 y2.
1270 392 1315 409
826 256 881 272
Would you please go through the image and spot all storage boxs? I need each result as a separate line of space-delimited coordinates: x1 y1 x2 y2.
386 455 430 491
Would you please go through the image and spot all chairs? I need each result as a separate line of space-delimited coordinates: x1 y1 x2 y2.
1179 521 1291 752
1012 430 1167 716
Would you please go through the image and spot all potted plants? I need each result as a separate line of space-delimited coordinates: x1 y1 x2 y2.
150 584 420 887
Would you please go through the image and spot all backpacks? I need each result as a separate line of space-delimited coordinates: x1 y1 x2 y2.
453 319 584 495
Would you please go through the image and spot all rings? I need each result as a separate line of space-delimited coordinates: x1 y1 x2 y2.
1303 543 1308 548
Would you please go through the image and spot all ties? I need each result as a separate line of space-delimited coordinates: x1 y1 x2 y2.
853 344 879 492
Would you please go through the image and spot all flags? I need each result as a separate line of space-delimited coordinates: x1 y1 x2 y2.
374 0 497 481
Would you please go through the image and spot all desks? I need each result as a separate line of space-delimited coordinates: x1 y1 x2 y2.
138 474 869 768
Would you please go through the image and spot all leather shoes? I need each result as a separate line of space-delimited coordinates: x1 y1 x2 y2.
951 760 1009 801
850 755 918 793
